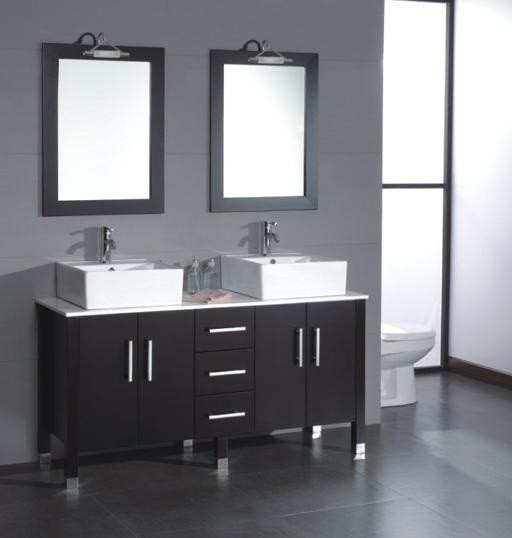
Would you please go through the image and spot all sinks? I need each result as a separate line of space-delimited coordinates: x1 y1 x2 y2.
55 259 184 309
220 252 348 300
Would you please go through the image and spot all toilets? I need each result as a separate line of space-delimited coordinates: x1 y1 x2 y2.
379 323 437 408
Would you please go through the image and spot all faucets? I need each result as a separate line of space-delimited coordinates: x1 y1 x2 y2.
98 225 117 262
257 218 281 256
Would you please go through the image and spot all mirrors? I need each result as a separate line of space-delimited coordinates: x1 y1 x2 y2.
40 30 167 217
208 39 321 214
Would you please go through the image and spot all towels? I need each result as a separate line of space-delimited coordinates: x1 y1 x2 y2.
182 290 232 304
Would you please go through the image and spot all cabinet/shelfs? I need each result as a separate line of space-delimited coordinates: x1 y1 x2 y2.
33 298 194 489
193 300 256 479
255 297 369 462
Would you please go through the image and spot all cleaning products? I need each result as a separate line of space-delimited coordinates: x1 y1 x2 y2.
184 254 223 294
